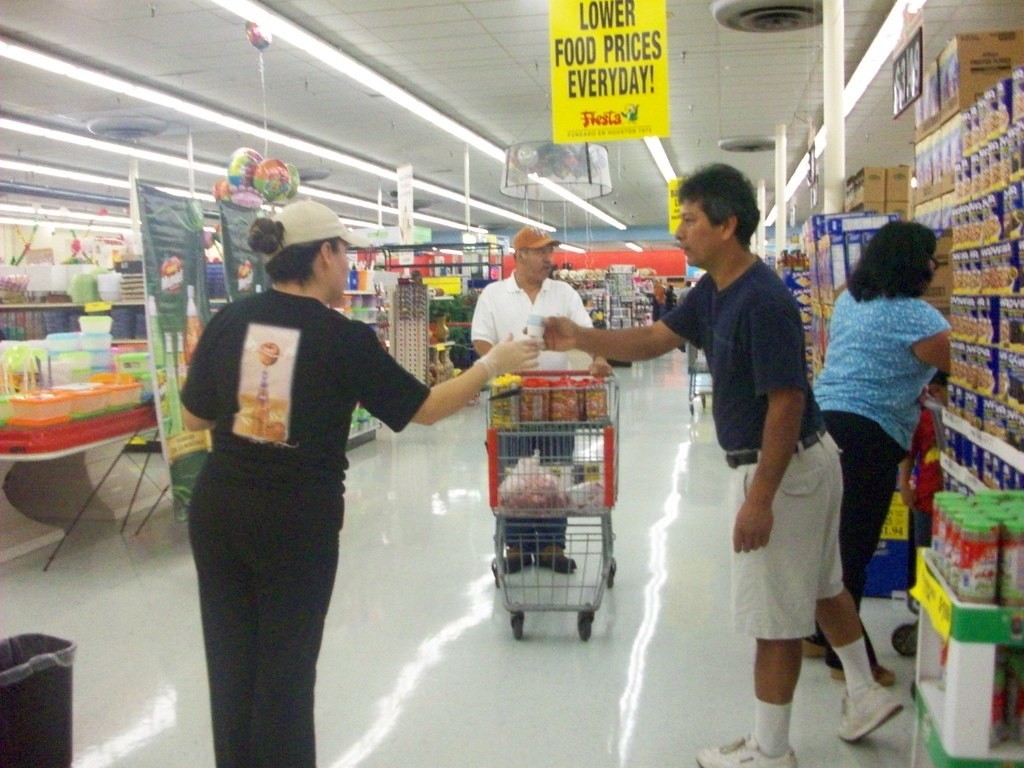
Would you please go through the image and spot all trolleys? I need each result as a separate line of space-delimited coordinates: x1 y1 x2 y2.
686 338 715 414
482 367 622 642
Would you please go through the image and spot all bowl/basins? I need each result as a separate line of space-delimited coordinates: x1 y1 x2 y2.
348 401 379 436
1 310 156 426
0 274 29 303
329 295 378 339
47 294 70 302
67 272 119 302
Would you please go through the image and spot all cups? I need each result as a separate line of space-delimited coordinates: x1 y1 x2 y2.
527 315 547 338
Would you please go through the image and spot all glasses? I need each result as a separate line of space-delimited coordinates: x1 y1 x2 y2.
930 255 941 271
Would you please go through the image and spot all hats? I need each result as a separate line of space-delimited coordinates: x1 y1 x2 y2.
258 199 383 265
514 226 561 251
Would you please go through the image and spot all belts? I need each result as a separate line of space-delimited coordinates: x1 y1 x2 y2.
726 422 826 469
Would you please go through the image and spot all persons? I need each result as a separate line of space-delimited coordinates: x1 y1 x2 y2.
525 164 904 768
180 199 543 768
805 220 952 687
901 367 950 544
472 226 612 572
647 280 691 320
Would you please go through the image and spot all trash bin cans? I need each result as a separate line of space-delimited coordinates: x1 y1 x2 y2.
0 632 78 768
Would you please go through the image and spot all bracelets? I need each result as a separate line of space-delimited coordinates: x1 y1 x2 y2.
473 356 499 383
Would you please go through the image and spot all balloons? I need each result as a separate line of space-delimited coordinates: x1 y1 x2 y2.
511 142 607 180
245 21 272 51
205 147 300 249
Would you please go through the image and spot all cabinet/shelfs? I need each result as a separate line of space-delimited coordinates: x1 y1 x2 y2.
911 546 1023 768
331 276 662 385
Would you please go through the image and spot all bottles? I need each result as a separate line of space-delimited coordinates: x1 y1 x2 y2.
147 283 173 437
346 264 375 292
492 373 607 429
931 490 1024 606
188 286 200 357
163 331 190 434
251 371 269 439
935 639 1024 747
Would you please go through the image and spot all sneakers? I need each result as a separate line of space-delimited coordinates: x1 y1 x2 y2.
504 545 531 573
534 545 576 572
696 734 798 768
838 680 903 743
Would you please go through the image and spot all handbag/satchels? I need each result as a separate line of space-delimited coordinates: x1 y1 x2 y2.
495 449 567 509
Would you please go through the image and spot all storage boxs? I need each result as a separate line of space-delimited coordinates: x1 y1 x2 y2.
844 28 1024 497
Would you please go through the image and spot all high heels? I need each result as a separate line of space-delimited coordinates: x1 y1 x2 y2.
800 635 828 658
830 663 894 686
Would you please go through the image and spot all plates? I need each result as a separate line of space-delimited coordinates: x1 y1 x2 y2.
120 274 145 300
